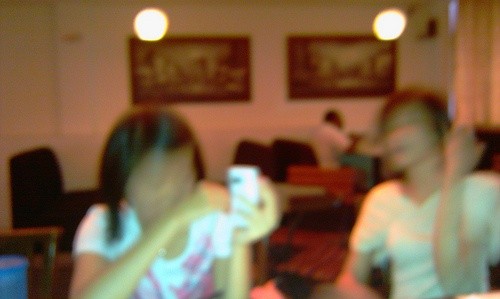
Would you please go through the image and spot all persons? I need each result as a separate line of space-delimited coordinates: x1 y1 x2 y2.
335 85 492 298
68 108 252 298
309 108 367 192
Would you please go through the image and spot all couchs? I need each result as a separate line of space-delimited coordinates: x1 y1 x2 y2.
233 139 387 230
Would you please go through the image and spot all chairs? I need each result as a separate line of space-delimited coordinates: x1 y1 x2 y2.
8 144 104 250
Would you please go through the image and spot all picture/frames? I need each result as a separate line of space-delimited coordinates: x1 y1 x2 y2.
283 32 398 100
124 33 255 106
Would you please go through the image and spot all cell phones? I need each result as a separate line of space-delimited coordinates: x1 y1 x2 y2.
226 164 260 229
